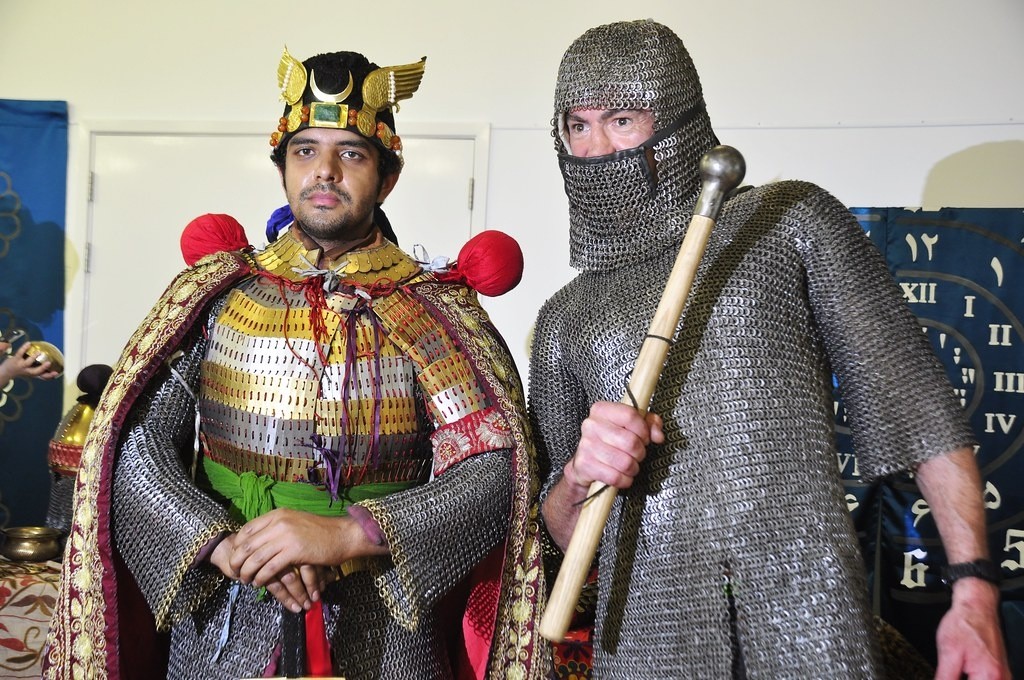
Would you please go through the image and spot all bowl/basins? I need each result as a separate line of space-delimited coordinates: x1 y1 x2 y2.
0 527 62 562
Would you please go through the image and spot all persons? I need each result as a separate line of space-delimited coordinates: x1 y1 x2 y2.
527 21 1014 679
48 49 548 680
1 338 59 388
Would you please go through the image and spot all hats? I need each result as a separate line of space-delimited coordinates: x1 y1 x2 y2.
266 51 427 176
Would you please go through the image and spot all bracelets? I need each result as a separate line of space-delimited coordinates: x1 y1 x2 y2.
939 558 1001 588
